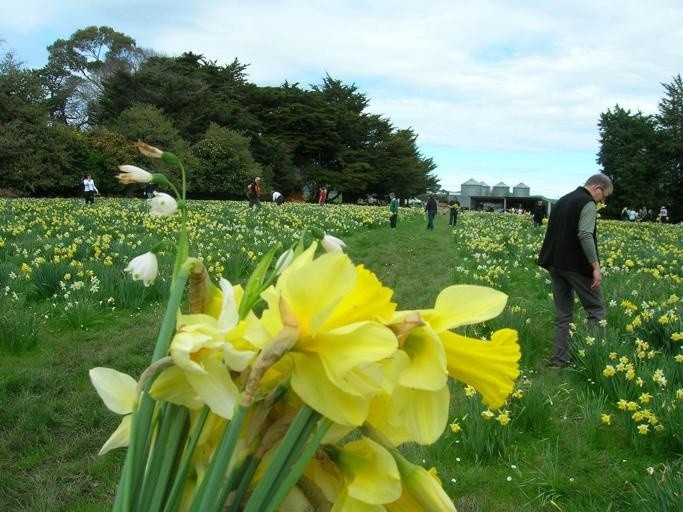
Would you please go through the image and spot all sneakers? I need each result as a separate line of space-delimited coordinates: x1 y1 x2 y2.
547 357 571 369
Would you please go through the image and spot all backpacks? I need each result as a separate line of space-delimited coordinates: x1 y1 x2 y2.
246 189 256 199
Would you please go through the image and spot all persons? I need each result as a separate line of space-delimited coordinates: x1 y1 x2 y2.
527 197 547 228
83 173 98 205
318 187 326 207
477 200 484 212
421 194 437 231
534 172 614 367
446 195 460 226
143 182 155 199
270 191 284 206
246 176 263 209
388 193 398 228
657 205 667 224
620 205 656 222
